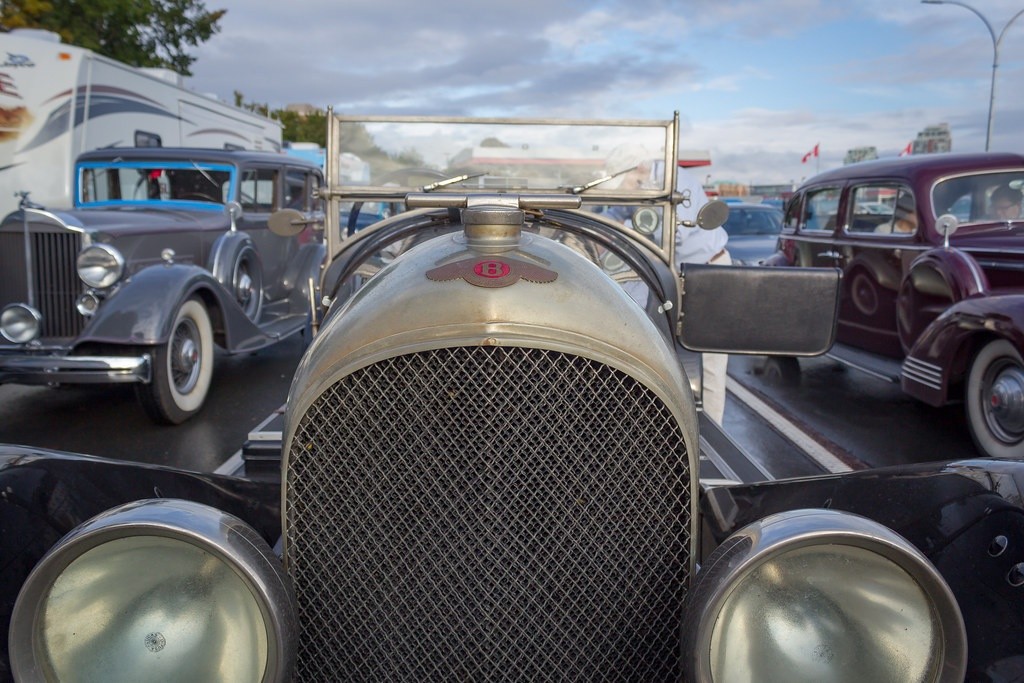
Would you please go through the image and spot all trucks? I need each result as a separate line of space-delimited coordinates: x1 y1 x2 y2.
0 28 285 214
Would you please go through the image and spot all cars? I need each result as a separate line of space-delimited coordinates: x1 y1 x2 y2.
761 153 1024 459
724 194 895 267
1 145 384 426
1 101 1024 683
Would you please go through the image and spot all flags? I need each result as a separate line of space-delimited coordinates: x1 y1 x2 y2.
899 143 910 157
800 142 818 164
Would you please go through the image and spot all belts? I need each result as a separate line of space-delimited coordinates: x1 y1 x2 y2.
710 250 725 262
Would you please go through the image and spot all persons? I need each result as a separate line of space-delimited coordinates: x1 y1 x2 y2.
987 186 1024 222
873 193 918 239
599 133 734 428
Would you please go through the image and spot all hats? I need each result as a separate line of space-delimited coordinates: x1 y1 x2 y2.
595 141 647 190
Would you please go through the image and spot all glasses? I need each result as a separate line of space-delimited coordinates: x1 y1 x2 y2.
987 203 1016 213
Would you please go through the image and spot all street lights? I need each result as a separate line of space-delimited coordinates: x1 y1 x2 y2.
919 0 1024 152
